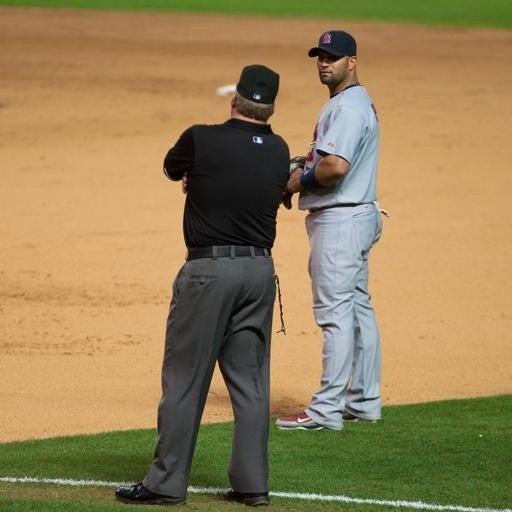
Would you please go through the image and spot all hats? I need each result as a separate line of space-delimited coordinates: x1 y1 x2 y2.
308 31 356 57
237 65 279 104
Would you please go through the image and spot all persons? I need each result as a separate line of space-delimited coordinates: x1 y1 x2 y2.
274 29 384 432
115 64 291 507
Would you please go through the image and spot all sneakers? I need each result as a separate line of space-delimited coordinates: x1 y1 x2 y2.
343 409 377 424
223 488 269 505
115 483 185 506
276 412 327 431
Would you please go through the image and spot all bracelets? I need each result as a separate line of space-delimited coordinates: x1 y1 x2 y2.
300 160 326 189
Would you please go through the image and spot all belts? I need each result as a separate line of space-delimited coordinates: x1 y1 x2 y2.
309 204 360 214
187 246 271 259
